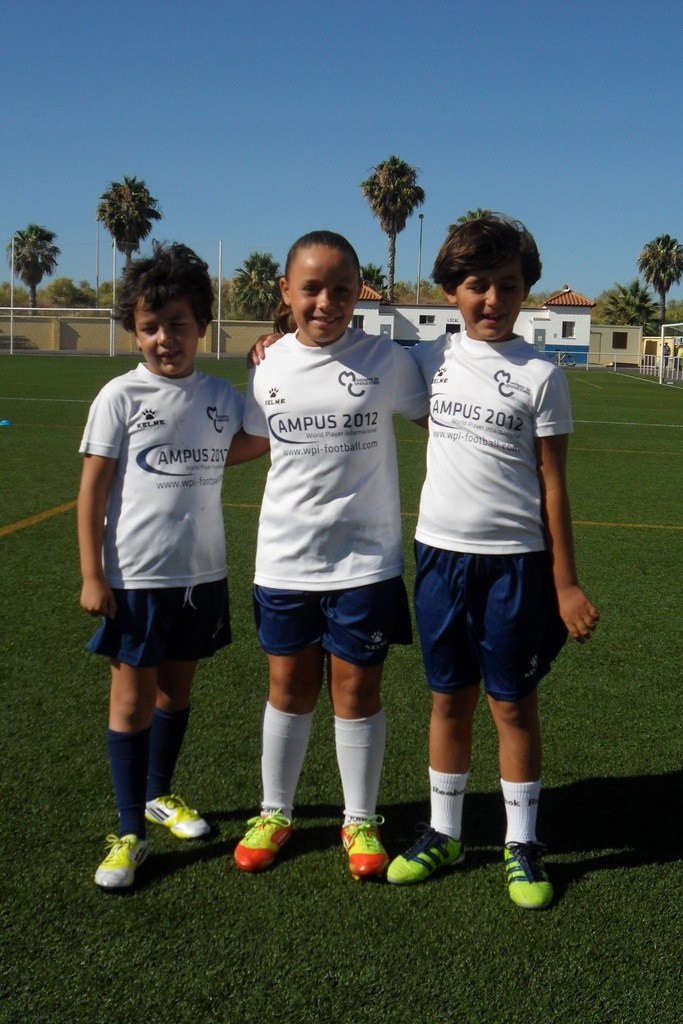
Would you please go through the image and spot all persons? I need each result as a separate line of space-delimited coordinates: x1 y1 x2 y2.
246 210 603 908
664 342 671 367
673 345 683 371
73 244 244 890
222 229 429 883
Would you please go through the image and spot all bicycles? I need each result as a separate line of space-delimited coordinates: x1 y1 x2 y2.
548 347 577 367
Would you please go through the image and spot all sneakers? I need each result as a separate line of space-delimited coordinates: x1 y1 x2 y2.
386 829 466 884
94 834 148 887
504 844 554 910
342 820 388 881
234 811 294 871
145 794 210 839
